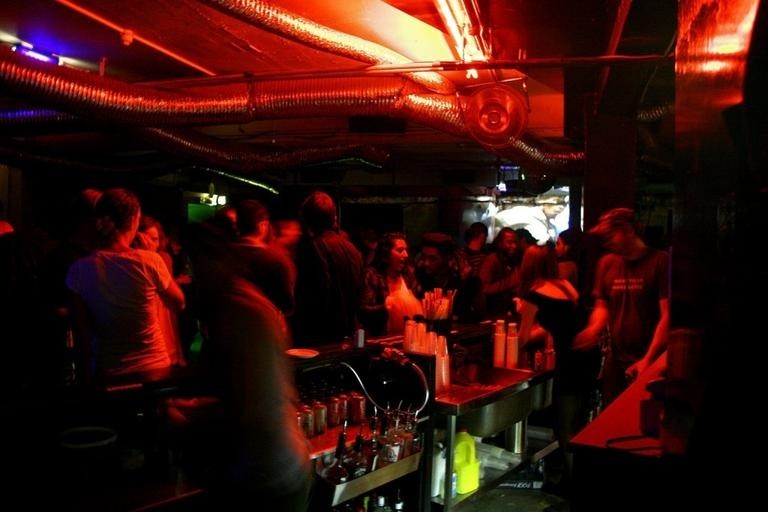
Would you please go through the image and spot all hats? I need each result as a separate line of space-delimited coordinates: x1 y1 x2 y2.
420 232 453 250
534 190 570 204
587 208 635 235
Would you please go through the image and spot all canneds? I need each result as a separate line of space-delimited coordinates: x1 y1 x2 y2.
296 392 367 439
543 349 557 371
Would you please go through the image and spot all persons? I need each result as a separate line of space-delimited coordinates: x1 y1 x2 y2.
1 179 766 512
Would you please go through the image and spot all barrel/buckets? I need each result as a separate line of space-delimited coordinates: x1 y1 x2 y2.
452 425 482 495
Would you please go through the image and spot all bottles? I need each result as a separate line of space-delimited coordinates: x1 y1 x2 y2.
61 326 79 389
348 488 407 512
335 401 420 478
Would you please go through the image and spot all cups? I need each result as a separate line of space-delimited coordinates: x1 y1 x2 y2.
493 320 519 369
404 319 451 395
